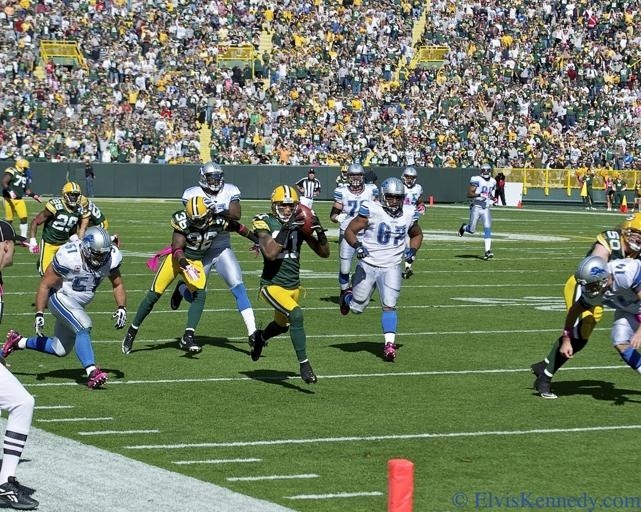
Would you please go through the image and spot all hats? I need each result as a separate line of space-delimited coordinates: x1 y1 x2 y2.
307 168 316 174
0 220 27 242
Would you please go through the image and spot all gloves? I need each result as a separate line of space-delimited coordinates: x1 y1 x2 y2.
8 190 17 200
29 238 39 255
405 198 412 204
334 213 348 222
401 247 416 262
310 210 327 246
275 210 304 247
204 194 218 209
33 312 45 339
354 241 369 259
481 191 488 197
418 203 426 215
112 306 128 329
186 264 201 281
493 197 498 207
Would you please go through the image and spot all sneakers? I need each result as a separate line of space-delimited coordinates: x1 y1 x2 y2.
340 289 351 316
300 361 318 384
88 367 107 389
20 241 29 248
383 341 396 361
484 250 494 258
179 335 202 353
404 267 413 280
459 223 466 237
10 479 35 494
0 481 39 511
249 330 264 362
114 233 119 247
171 279 185 311
2 328 22 357
122 334 133 356
530 361 545 375
535 373 558 400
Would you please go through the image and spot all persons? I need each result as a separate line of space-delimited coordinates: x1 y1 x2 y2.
170 162 269 349
0 219 69 510
121 197 261 356
493 171 507 206
1 159 43 248
336 165 350 188
399 167 426 280
602 174 616 211
330 164 380 309
1 226 127 390
458 163 497 261
613 174 628 211
295 168 322 209
584 169 596 210
559 256 641 378
339 177 423 362
29 182 91 310
248 185 330 385
631 179 641 212
76 195 120 249
84 160 96 198
577 174 587 204
530 213 641 399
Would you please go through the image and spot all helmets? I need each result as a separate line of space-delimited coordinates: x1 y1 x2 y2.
479 164 491 181
62 182 82 208
269 185 299 222
81 227 112 267
198 162 224 193
187 196 215 231
402 168 418 188
347 165 364 187
16 159 30 174
341 164 349 180
574 257 609 297
380 178 404 216
623 212 640 251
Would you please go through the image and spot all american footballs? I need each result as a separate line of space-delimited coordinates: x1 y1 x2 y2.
294 203 315 237
